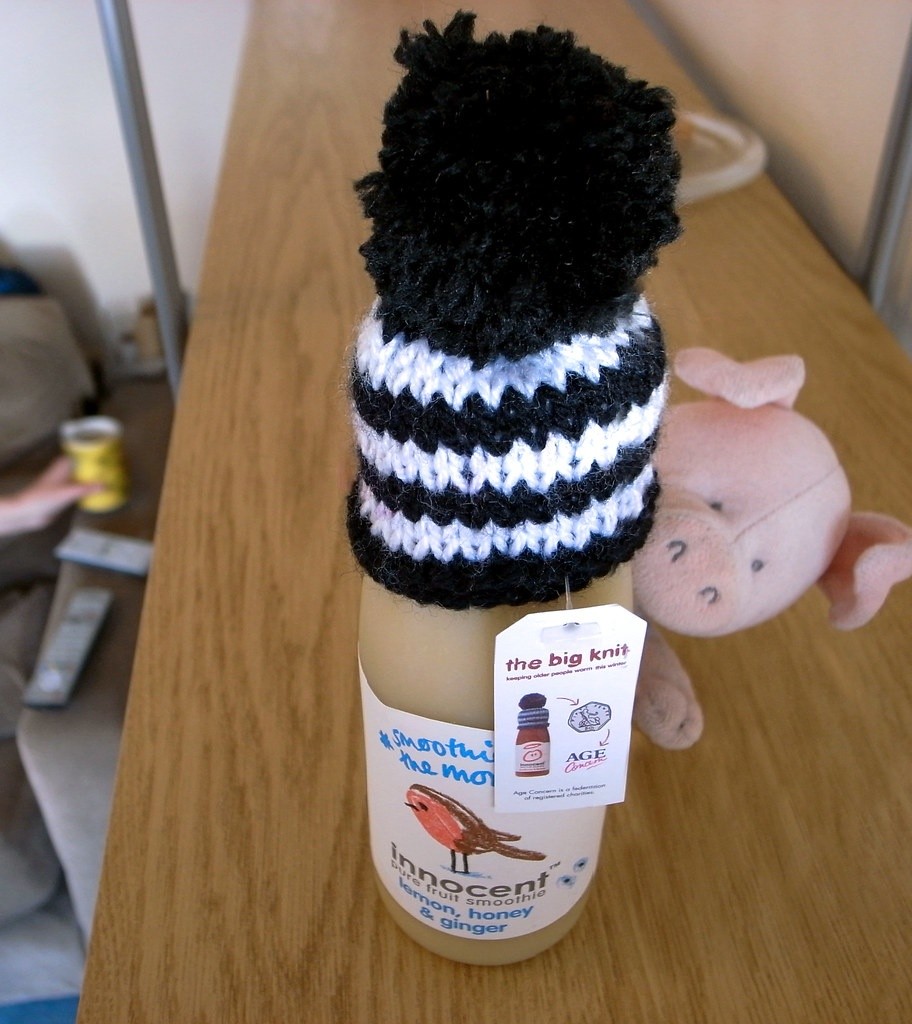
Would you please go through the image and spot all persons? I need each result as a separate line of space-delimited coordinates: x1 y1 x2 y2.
0 453 116 539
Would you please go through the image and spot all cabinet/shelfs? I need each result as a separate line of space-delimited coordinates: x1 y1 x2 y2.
77 0 912 1024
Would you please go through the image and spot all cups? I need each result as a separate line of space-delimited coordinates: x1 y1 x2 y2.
60 419 127 514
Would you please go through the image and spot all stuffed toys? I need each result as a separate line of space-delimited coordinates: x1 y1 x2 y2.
628 348 912 750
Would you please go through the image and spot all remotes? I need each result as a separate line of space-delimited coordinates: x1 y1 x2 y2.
22 588 112 707
53 526 154 577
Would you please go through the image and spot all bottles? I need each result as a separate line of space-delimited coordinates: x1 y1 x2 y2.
361 572 639 965
513 686 551 775
